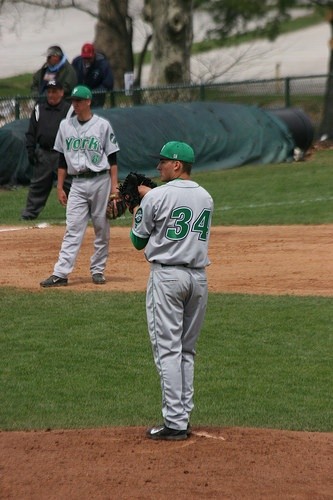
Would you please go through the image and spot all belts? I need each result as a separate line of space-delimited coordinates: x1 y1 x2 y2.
71 170 107 178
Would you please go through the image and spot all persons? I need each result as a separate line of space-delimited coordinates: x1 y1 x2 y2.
71 43 114 108
125 141 214 441
39 86 119 287
33 46 78 105
20 81 77 221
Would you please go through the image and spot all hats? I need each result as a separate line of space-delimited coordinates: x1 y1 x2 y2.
43 79 64 88
81 44 94 57
47 46 63 61
66 85 92 101
149 141 195 165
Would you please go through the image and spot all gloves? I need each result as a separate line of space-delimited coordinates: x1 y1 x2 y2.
28 148 39 166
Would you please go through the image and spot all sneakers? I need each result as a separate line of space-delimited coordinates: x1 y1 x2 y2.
40 275 67 287
93 273 105 283
146 422 191 440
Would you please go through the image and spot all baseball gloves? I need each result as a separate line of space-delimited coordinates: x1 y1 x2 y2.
117 170 157 214
107 191 127 220
